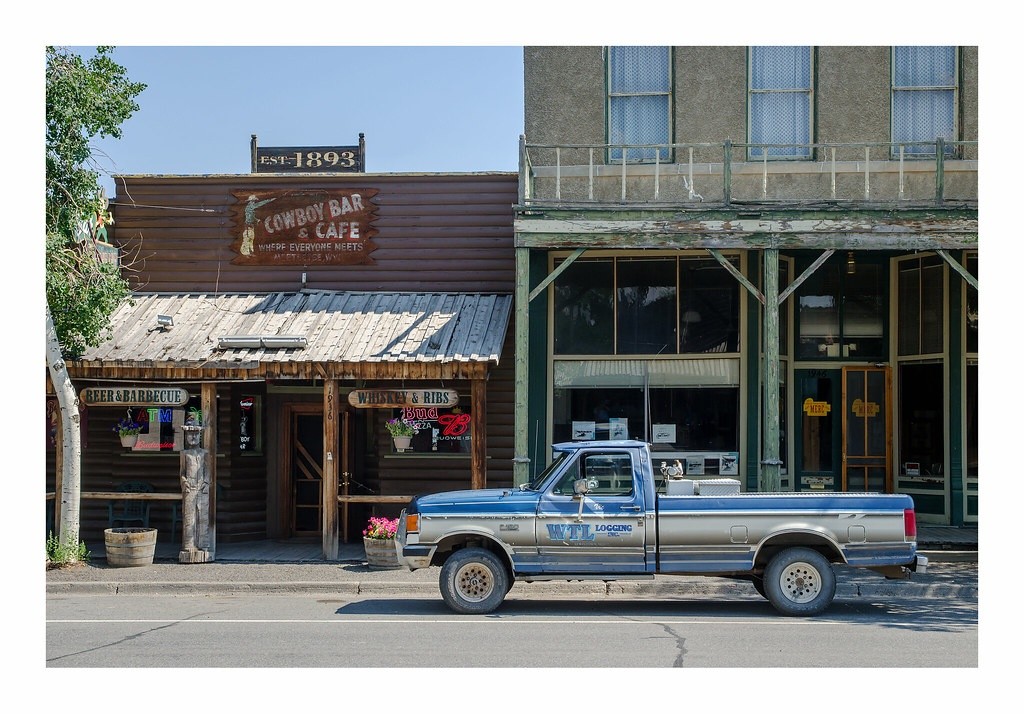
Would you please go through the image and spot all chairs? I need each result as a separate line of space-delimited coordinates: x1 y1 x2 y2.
108 480 154 528
171 482 224 545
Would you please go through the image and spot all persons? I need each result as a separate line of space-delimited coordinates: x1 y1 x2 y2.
179 415 212 553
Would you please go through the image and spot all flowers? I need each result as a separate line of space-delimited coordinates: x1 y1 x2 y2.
362 517 399 539
385 418 421 439
112 419 143 438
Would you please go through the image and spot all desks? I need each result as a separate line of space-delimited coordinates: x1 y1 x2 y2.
666 479 694 495
694 478 742 495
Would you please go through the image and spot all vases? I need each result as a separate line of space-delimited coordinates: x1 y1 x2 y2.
393 436 411 449
119 435 138 447
363 535 403 569
104 528 158 566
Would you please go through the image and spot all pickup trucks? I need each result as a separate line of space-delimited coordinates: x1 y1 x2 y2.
394 436 929 615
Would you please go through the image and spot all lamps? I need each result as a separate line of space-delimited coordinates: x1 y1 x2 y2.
157 314 174 329
218 335 308 349
681 309 702 346
847 251 855 274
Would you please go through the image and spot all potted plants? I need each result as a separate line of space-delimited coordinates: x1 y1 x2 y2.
180 407 205 446
